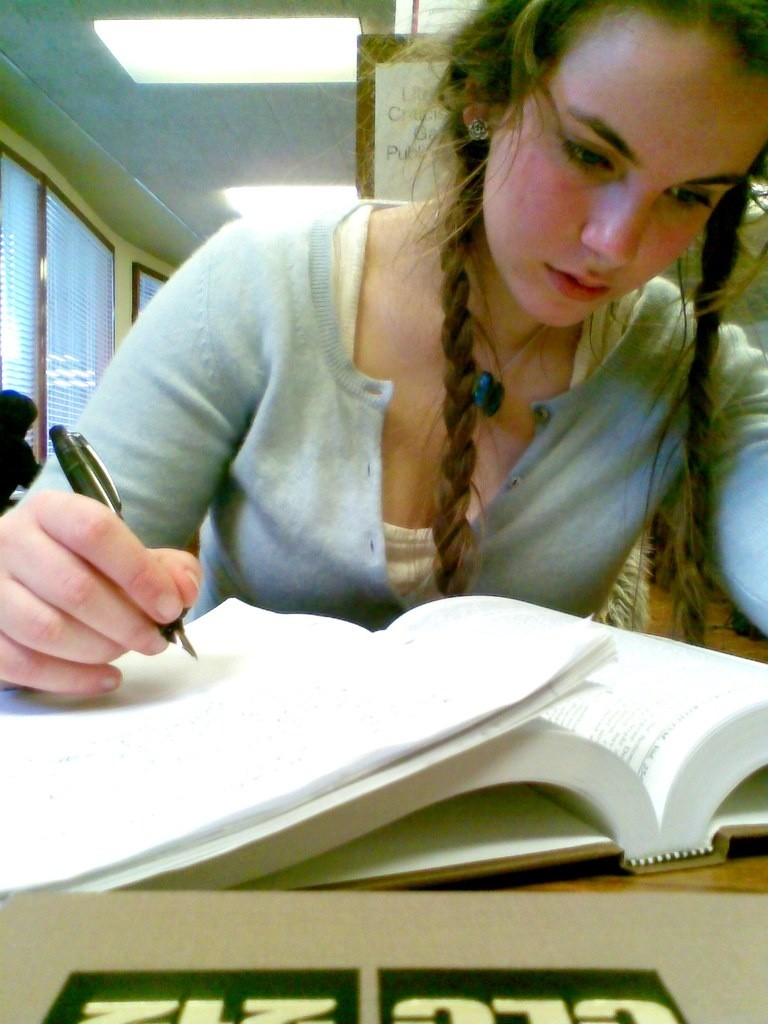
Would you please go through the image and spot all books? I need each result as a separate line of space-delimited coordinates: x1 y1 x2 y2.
0 594 768 892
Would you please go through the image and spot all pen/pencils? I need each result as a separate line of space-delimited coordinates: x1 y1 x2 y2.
49 424 199 659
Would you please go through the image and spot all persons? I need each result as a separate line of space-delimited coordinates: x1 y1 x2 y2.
0 1 768 699
0 387 43 515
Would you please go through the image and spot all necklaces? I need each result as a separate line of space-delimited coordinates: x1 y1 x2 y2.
433 193 549 418
